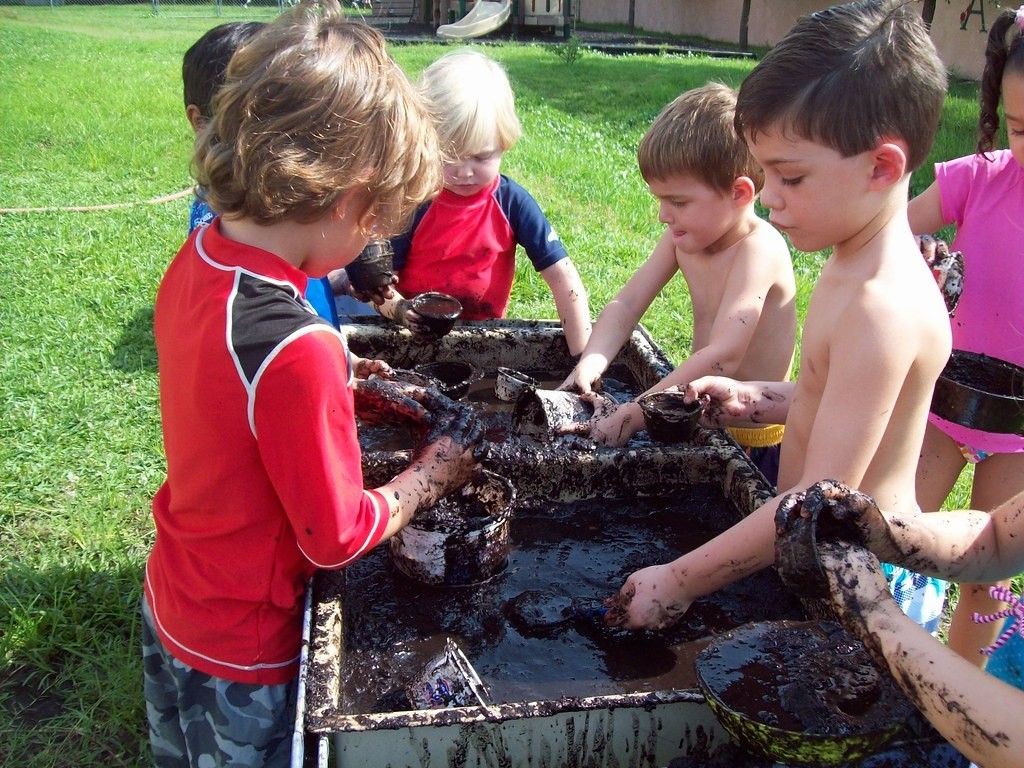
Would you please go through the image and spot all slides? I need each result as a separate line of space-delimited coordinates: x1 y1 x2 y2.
436 0 513 39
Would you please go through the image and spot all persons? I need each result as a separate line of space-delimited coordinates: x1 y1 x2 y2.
182 0 1024 768
143 0 489 768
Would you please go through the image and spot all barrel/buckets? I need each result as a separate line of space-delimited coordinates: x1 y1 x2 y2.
390 468 515 585
510 386 619 440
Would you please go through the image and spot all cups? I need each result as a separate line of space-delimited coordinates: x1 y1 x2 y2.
369 637 491 715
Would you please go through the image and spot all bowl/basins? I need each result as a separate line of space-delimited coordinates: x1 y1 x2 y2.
774 508 867 599
413 292 463 337
638 392 705 445
412 360 484 400
494 367 540 400
695 620 908 768
